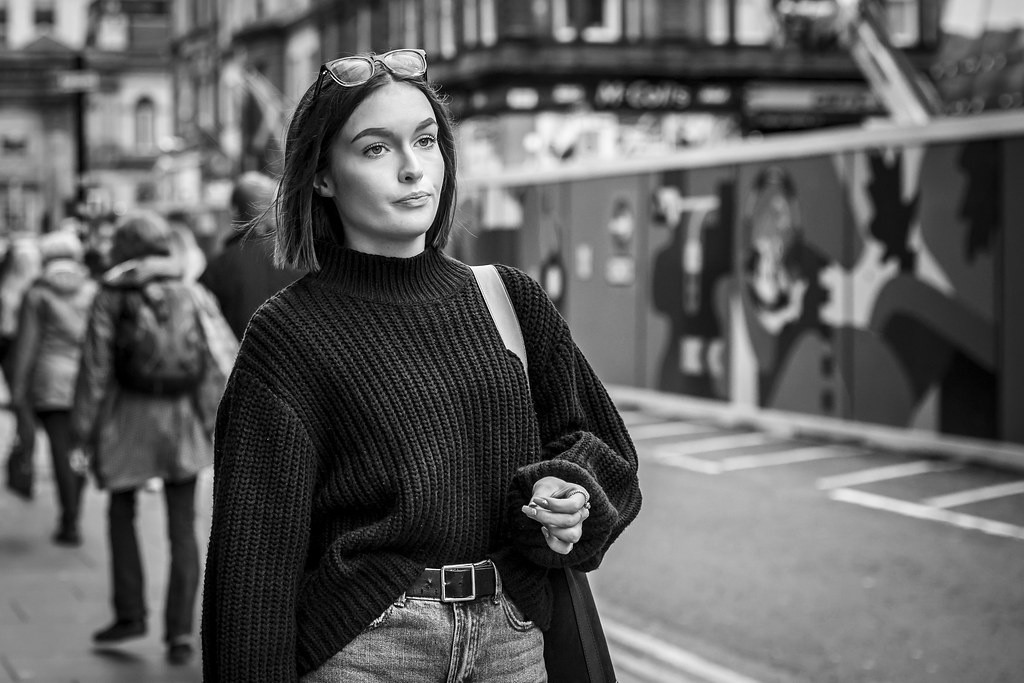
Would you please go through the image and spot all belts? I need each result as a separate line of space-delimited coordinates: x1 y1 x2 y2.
409 564 495 603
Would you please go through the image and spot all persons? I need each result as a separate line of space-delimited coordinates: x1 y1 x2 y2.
199 48 642 683
70 218 220 666
0 172 314 546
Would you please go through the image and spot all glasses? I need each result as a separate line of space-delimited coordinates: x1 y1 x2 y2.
313 49 428 100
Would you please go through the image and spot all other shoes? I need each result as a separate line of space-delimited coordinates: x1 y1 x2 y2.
168 644 194 664
54 529 82 547
93 616 147 645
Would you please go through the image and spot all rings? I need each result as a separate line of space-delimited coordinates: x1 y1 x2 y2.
577 510 585 524
567 490 591 509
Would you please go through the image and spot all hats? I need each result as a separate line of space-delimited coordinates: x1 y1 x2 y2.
37 228 84 264
111 213 175 254
230 172 277 218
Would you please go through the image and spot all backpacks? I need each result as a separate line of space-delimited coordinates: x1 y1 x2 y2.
107 279 209 395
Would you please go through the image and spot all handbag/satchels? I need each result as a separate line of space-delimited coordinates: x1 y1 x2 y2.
539 567 615 683
3 446 33 496
